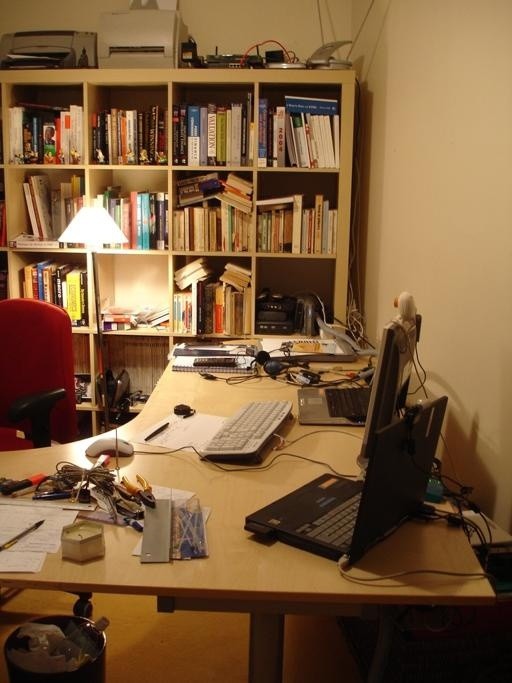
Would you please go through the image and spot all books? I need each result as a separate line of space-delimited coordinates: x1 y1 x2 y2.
176 172 222 207
256 194 336 255
219 262 251 292
215 172 253 215
174 257 216 291
23 260 89 327
8 103 84 164
91 105 168 166
10 232 80 249
258 96 340 169
144 306 169 328
170 343 257 373
173 96 254 167
23 174 84 248
173 283 251 336
91 185 168 251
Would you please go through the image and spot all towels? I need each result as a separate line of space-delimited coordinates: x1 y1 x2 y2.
4 614 106 683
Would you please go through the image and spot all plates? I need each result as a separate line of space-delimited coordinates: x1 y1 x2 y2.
0 69 362 438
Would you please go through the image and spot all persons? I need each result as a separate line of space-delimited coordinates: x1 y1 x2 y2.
45 127 55 145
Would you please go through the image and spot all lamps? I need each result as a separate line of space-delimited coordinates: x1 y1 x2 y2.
1 299 82 453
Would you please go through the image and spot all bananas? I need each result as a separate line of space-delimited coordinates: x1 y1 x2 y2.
198 401 293 466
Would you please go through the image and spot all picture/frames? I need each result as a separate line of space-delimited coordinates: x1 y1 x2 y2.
0 336 499 682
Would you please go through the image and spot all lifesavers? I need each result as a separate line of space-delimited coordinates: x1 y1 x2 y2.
401 583 512 641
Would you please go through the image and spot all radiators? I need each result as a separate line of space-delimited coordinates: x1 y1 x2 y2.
193 358 235 367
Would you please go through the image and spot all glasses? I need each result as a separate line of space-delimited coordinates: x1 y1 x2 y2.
58 207 128 431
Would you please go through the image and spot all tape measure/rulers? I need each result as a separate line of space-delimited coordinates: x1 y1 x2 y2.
122 473 156 508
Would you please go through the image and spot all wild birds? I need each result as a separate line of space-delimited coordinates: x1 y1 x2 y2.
245 394 449 570
298 370 376 427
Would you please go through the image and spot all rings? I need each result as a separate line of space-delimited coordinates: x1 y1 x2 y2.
33 490 72 501
1 519 45 553
11 486 35 497
145 423 169 441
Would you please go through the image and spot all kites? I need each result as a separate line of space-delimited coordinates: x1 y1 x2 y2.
124 517 143 532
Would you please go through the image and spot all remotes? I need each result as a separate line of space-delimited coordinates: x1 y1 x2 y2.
85 437 134 458
298 370 320 384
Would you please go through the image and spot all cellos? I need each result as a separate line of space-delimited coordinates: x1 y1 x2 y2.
97 8 190 70
0 30 97 69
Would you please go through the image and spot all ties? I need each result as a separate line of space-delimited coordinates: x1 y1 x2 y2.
357 313 421 475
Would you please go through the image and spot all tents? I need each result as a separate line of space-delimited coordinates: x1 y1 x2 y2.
257 347 298 374
